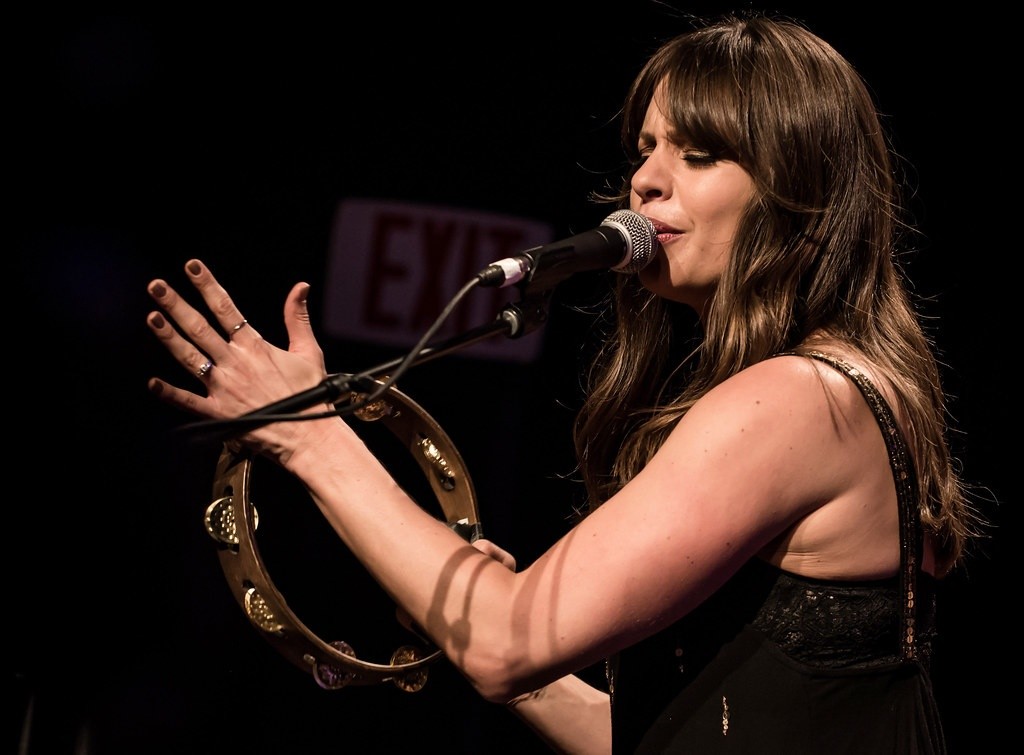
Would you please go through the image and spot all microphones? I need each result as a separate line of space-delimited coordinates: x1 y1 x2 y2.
476 210 659 290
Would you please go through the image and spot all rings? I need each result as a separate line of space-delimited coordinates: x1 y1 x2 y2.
230 320 248 334
196 361 212 376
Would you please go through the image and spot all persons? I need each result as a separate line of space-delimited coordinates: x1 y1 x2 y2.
144 13 968 755
